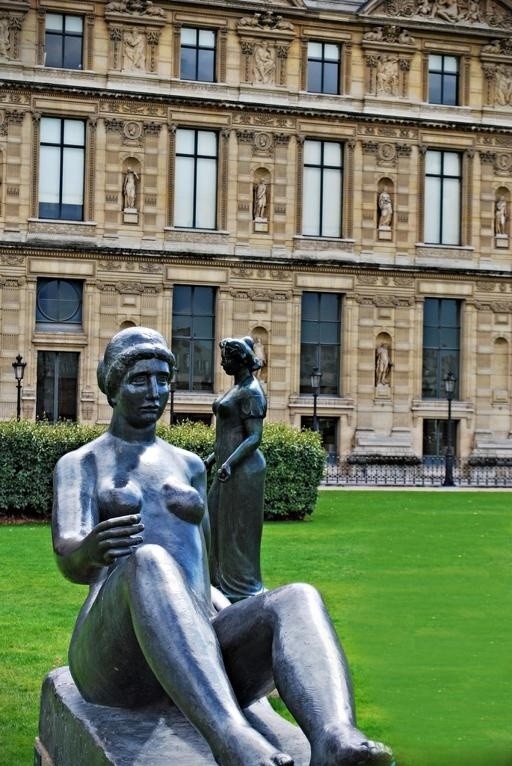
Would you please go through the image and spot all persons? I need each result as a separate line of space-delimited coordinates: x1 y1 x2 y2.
255 177 267 219
497 194 508 234
122 166 140 208
253 335 266 379
376 340 390 385
495 209 504 234
372 52 401 95
51 324 394 765
494 64 511 105
202 335 268 605
378 186 393 225
124 28 142 69
253 39 275 83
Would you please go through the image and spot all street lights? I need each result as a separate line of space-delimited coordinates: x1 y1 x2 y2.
170 365 179 427
11 354 27 423
310 366 322 433
442 370 456 488
